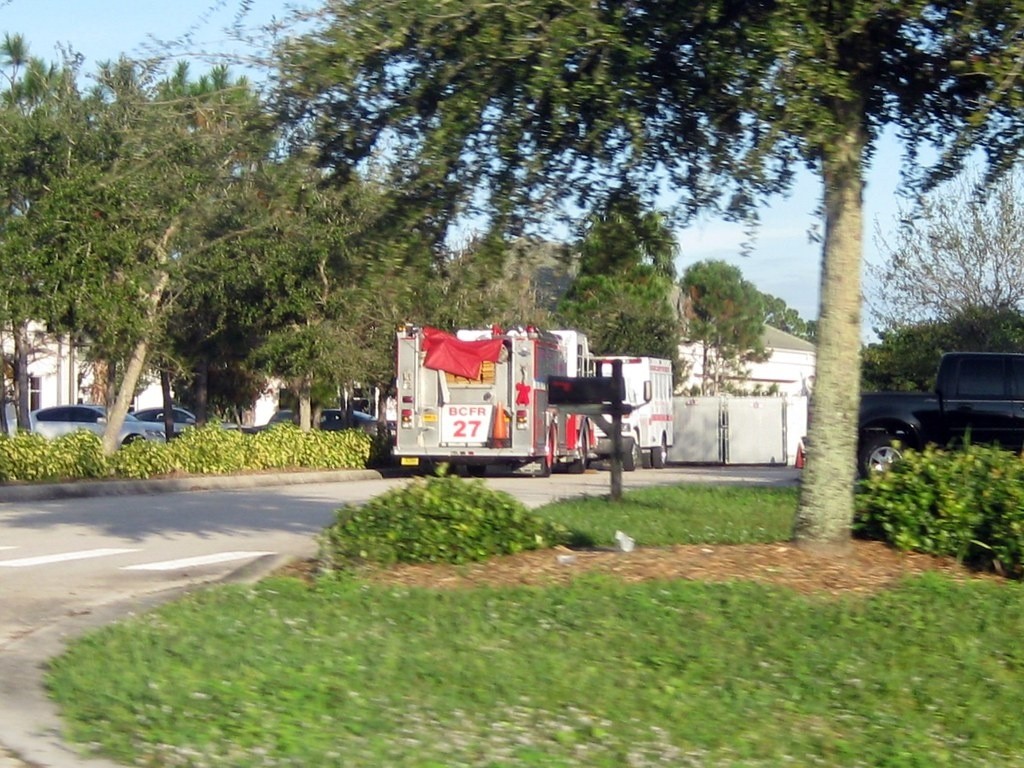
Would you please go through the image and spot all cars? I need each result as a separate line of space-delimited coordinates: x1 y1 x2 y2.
131 406 252 439
246 408 397 448
6 403 167 450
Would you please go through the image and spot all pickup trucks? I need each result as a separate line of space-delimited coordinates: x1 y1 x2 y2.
855 350 1024 479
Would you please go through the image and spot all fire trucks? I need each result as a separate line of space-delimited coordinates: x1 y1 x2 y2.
389 319 677 478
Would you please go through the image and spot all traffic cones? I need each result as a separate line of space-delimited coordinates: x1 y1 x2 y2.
794 443 805 469
